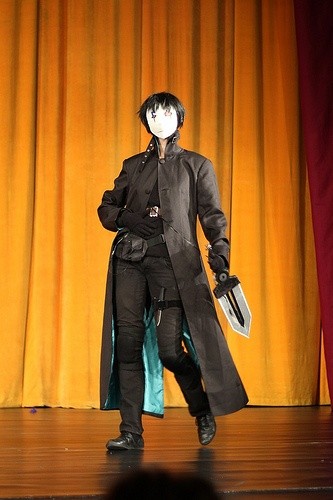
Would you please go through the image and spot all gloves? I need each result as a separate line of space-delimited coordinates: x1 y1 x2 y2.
123 208 157 238
209 254 226 273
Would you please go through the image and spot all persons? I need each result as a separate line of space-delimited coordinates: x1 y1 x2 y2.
97 91 230 449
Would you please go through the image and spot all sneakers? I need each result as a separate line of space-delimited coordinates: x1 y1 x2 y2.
107 431 144 451
196 415 216 445
